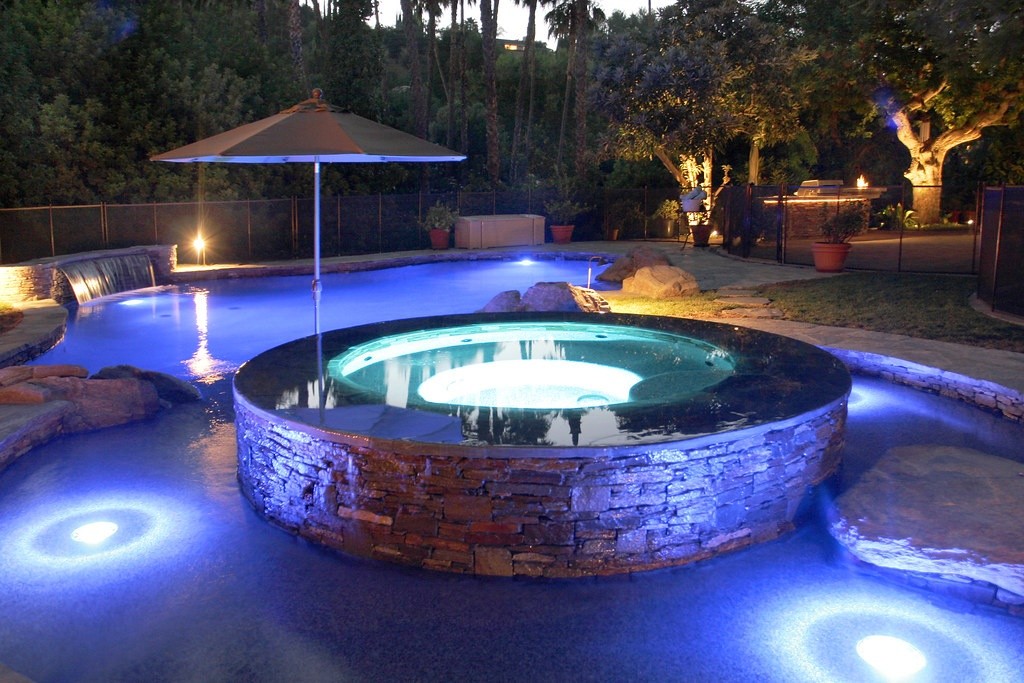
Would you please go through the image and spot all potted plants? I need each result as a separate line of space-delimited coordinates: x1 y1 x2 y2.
810 205 865 273
688 205 713 246
543 198 592 244
654 199 679 239
416 200 458 250
598 199 644 241
